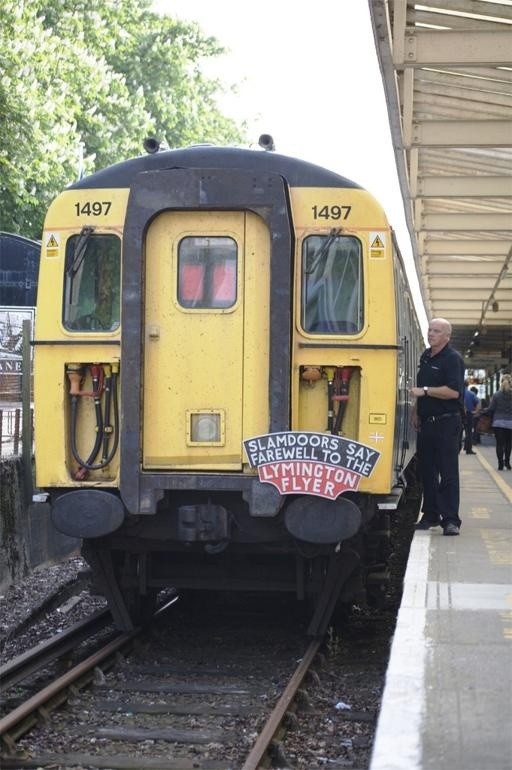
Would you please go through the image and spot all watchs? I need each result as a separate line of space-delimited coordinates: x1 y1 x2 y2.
423 386 428 396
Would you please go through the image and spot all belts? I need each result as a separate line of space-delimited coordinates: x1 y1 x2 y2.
426 412 461 424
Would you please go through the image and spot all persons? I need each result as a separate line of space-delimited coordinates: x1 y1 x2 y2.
410 317 466 534
462 373 512 472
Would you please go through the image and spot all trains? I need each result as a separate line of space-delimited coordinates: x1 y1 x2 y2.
34 133 427 641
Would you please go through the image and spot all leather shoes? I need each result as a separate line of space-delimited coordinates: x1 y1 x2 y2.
499 463 504 470
467 449 476 454
443 522 460 535
411 512 442 529
505 463 512 470
